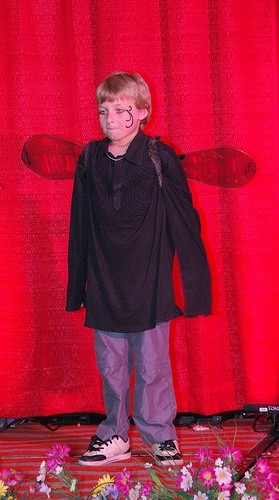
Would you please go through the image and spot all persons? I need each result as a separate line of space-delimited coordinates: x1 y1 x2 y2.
65 71 211 467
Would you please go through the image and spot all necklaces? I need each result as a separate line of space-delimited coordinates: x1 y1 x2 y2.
106 153 123 162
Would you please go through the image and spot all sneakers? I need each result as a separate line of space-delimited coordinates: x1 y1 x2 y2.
151 439 184 466
77 435 132 466
